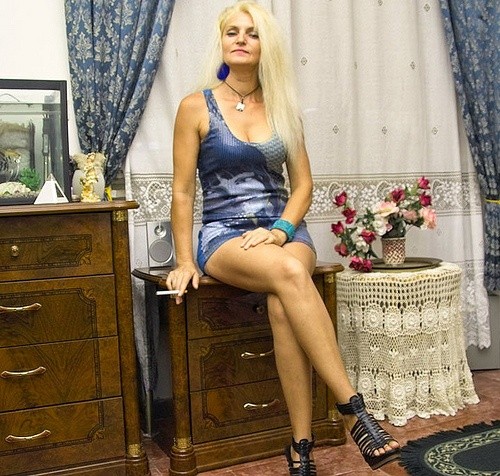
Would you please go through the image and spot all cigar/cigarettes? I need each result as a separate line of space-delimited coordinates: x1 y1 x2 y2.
156 290 188 295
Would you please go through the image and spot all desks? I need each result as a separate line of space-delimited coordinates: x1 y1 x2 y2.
336 258 481 426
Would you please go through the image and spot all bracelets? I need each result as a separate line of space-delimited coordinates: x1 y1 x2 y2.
270 219 296 242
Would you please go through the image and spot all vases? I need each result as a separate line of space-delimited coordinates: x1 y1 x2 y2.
381 237 407 265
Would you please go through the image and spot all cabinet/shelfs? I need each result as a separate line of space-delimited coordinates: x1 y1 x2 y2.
0 195 150 476
133 261 339 476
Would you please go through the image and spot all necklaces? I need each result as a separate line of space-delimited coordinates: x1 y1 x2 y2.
224 81 261 112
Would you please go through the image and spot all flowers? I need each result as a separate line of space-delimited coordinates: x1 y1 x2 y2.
328 174 435 276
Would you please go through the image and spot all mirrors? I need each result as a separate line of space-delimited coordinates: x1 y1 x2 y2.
0 78 70 206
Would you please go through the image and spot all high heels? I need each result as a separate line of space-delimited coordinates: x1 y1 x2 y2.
286 433 318 476
335 393 401 470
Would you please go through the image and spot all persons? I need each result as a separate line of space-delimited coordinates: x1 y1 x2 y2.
80 153 98 199
165 0 402 476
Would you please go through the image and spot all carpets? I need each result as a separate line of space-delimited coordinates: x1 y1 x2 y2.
397 420 500 476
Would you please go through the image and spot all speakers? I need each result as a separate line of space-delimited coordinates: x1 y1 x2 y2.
146 221 175 268
